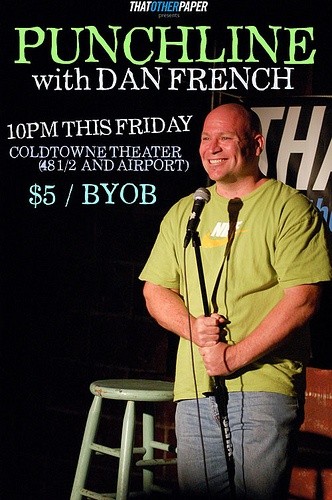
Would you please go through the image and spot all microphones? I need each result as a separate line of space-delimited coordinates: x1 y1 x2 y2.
183 188 211 248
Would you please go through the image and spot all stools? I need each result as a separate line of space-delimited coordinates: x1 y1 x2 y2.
70 379 176 500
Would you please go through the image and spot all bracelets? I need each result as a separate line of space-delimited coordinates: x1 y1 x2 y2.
222 344 237 375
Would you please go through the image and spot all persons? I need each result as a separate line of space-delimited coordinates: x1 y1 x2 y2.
137 101 332 500
